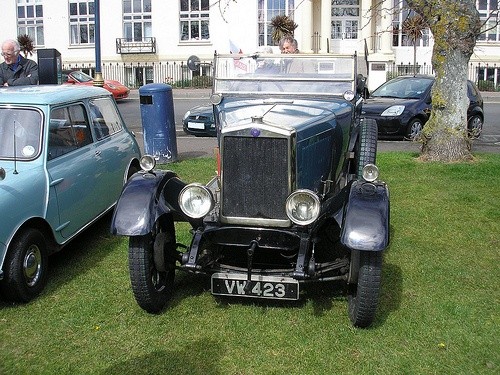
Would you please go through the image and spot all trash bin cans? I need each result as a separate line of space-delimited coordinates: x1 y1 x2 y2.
139 83 179 164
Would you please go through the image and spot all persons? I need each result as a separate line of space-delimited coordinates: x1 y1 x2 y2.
0 39 39 87
279 36 302 54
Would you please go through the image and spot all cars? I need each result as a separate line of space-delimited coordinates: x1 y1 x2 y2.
182 102 216 136
361 75 484 141
110 49 391 329
0 85 141 302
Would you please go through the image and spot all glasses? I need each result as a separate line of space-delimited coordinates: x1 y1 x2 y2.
1 51 16 56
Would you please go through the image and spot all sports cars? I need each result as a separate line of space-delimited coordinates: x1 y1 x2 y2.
62 70 130 101
